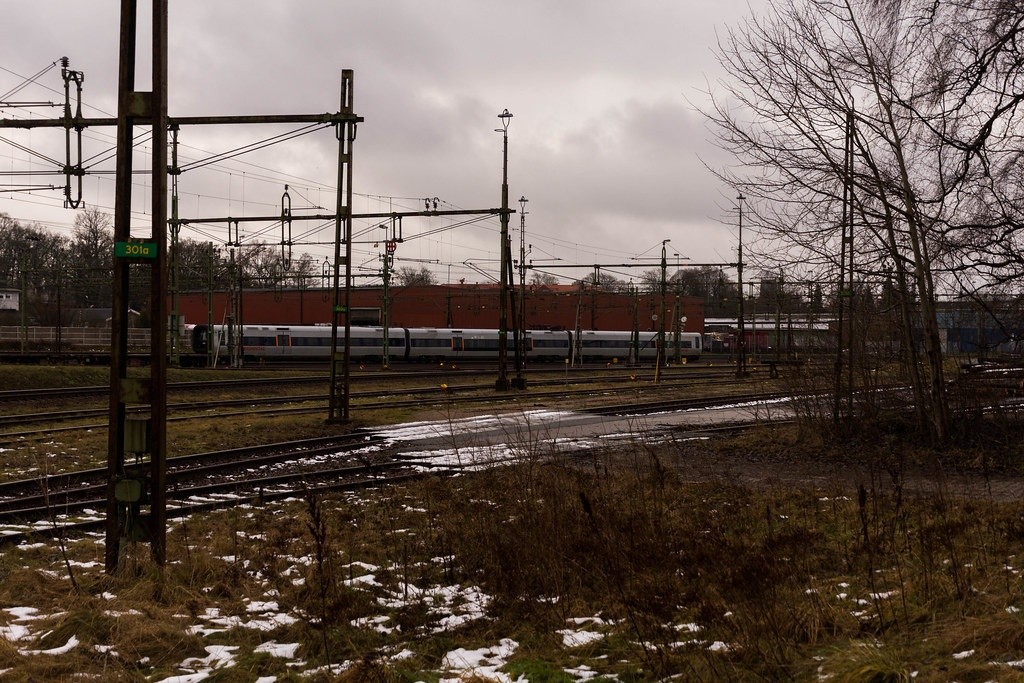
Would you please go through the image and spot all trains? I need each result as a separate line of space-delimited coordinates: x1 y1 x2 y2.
194 325 705 364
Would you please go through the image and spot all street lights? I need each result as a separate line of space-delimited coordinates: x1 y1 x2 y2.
493 108 517 394
736 193 746 379
512 195 530 389
